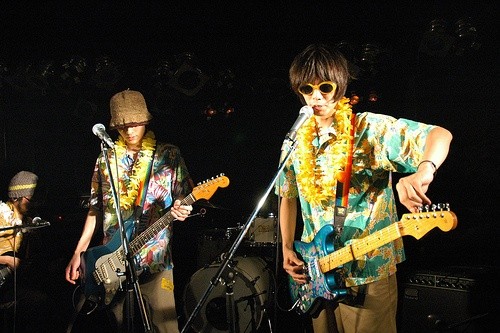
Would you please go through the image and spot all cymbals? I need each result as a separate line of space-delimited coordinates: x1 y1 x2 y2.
193 200 229 211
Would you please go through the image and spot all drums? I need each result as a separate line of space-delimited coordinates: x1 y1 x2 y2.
196 228 232 267
183 252 276 333
236 211 282 249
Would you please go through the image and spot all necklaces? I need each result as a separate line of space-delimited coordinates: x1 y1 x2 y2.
109 136 156 210
297 98 354 206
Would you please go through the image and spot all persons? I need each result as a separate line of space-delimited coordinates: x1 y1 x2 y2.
275 43 454 333
0 171 39 274
65 88 193 333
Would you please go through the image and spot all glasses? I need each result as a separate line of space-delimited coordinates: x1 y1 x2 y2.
24 197 37 206
297 82 337 95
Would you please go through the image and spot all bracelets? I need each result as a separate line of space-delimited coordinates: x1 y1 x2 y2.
417 160 436 170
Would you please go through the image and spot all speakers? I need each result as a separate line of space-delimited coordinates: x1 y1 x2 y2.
395 275 500 333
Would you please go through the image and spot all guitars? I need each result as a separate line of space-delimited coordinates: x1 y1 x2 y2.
288 203 458 317
75 173 231 310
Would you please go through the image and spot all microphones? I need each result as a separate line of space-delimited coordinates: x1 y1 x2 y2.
281 105 314 151
31 216 50 226
92 123 114 149
427 314 443 329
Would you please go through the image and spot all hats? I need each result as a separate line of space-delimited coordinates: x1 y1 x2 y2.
109 88 151 129
8 171 42 198
